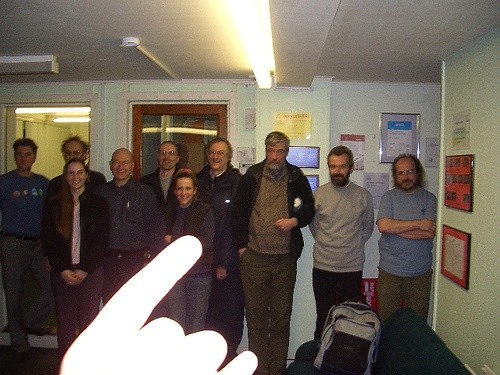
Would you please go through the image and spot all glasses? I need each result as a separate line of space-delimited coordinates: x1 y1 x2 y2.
65 169 86 178
173 186 193 195
267 147 286 157
207 149 227 159
158 149 177 158
111 159 131 166
329 162 348 171
63 149 82 157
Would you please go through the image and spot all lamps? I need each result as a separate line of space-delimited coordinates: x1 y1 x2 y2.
0 55 60 74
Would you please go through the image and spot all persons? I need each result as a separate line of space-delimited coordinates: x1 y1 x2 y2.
233 131 316 375
194 138 245 373
102 148 167 306
165 168 216 337
142 140 184 210
309 145 374 341
42 136 108 274
0 138 54 369
374 153 438 326
42 158 111 375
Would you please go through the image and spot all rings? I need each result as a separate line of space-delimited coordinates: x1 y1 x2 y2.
68 282 72 285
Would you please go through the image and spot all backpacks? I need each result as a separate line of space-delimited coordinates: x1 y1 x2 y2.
311 299 382 375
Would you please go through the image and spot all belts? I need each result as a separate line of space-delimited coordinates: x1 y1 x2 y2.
0 230 41 242
106 247 132 259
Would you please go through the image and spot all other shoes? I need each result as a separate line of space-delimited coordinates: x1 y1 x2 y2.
24 324 52 337
14 349 30 364
